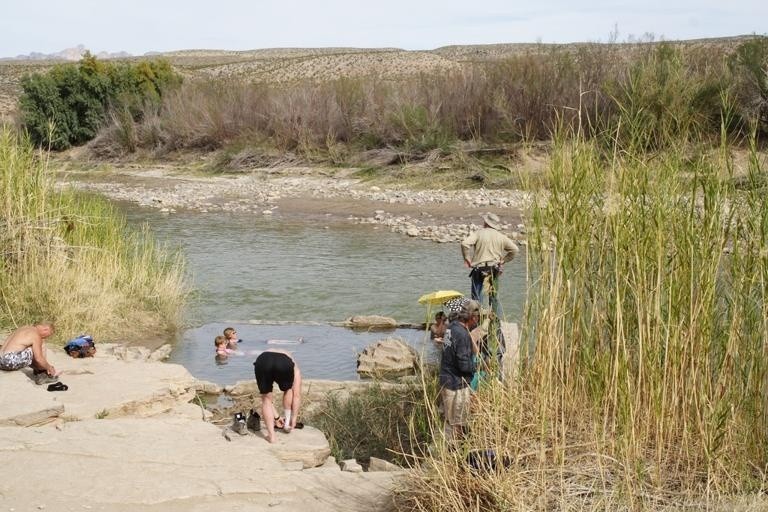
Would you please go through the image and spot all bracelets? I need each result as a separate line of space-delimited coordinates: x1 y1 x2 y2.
273 415 281 420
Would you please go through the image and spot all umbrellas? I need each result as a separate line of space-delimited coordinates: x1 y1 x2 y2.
417 290 463 304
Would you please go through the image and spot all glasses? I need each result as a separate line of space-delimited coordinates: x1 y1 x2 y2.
232 331 236 334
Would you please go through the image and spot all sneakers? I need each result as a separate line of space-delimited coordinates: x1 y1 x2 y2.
231 409 260 435
47 382 68 391
294 422 304 430
35 373 59 385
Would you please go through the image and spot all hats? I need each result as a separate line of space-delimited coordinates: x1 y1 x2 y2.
482 213 502 231
460 300 489 316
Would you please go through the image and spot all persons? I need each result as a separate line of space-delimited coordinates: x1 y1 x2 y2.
214 334 234 356
461 211 520 322
440 299 497 455
254 347 302 442
444 296 508 393
430 311 448 347
0 319 60 385
223 327 242 343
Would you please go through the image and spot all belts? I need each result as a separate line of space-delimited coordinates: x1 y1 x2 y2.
477 262 498 267
